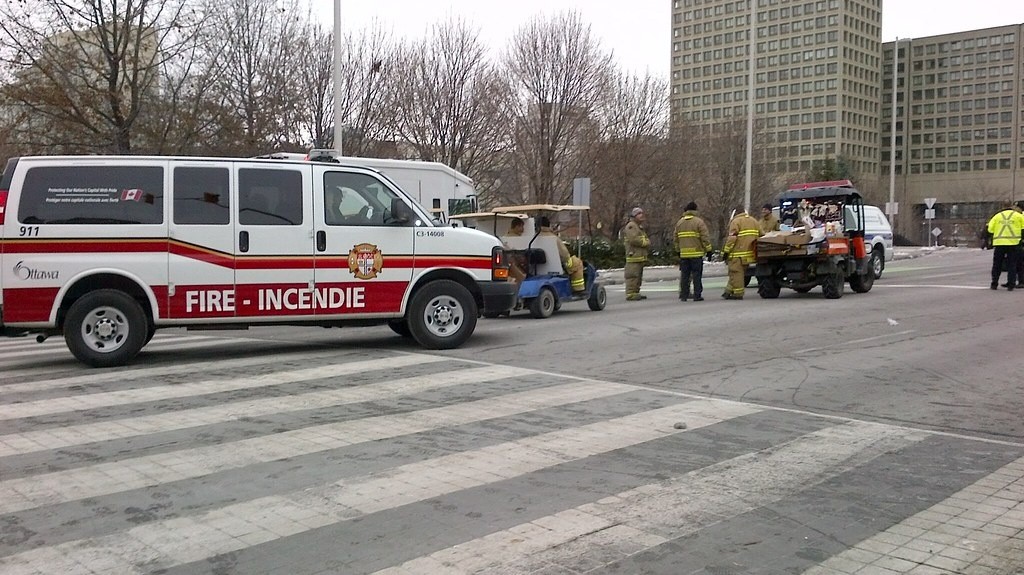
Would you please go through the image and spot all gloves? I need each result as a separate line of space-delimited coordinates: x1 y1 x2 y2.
677 252 680 257
706 251 714 262
722 253 729 265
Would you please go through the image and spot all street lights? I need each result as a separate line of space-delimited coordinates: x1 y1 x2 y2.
889 37 913 233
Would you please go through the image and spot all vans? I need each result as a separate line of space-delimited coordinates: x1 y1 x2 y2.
0 148 520 370
771 205 895 279
245 152 482 234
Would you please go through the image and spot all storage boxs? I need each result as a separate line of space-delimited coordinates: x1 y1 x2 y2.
785 224 811 244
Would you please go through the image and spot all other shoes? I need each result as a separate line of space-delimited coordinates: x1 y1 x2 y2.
640 295 647 299
722 291 734 297
572 290 589 296
1007 286 1015 291
693 297 704 301
1015 283 1024 288
1000 283 1016 287
990 285 997 290
725 294 744 299
687 292 694 299
680 298 687 301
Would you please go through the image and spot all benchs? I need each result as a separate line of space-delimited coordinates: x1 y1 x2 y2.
500 236 566 276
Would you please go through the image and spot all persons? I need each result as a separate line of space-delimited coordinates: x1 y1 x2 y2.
507 217 585 297
721 205 780 300
673 203 713 301
325 186 370 226
981 198 1024 291
624 207 650 301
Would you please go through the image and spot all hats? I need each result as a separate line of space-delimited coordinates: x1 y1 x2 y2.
762 203 772 211
731 204 744 211
1012 200 1024 206
686 202 697 210
631 207 643 216
